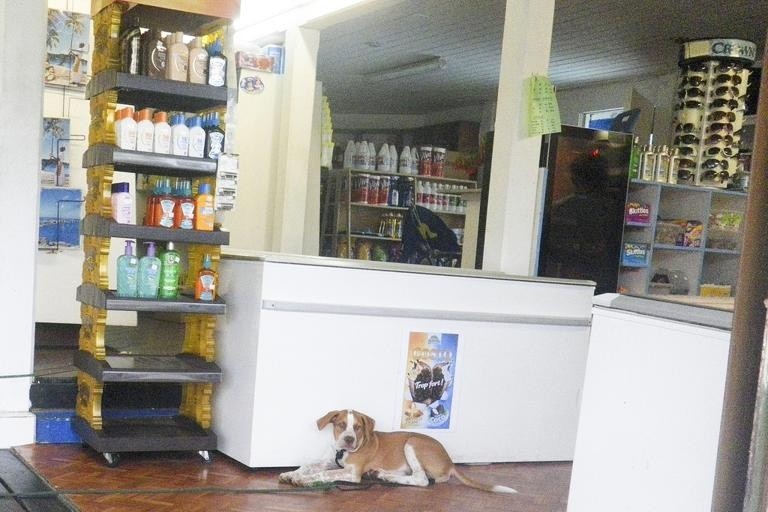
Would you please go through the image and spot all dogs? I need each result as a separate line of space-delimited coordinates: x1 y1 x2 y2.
277 408 520 496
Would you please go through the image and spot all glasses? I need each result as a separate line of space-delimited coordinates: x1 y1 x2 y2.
672 159 697 169
673 135 701 146
671 170 694 180
706 110 737 123
714 61 744 73
675 87 705 99
712 86 740 99
704 134 734 146
708 99 740 111
678 62 708 76
674 146 697 157
674 122 701 134
675 100 704 111
701 158 729 170
676 76 706 89
706 122 734 134
713 74 743 86
700 170 730 181
729 68 754 179
703 145 732 158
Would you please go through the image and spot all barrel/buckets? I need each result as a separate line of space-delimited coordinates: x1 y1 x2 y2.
321 139 470 262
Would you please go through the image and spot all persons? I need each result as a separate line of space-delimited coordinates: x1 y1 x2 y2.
549 152 623 296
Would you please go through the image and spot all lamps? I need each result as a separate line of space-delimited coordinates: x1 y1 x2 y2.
368 55 449 83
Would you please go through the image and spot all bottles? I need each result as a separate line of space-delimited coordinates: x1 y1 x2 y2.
336 141 469 268
116 103 226 163
195 254 219 302
113 174 216 232
160 239 180 303
120 11 226 86
631 132 681 187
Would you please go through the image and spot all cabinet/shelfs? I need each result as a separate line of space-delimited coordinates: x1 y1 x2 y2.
320 168 481 266
616 176 747 294
76 0 240 466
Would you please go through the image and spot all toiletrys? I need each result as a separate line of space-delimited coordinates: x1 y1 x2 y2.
110 15 227 302
625 136 680 183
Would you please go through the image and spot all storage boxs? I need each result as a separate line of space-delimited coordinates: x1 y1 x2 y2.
700 281 733 298
621 240 651 265
624 200 651 223
